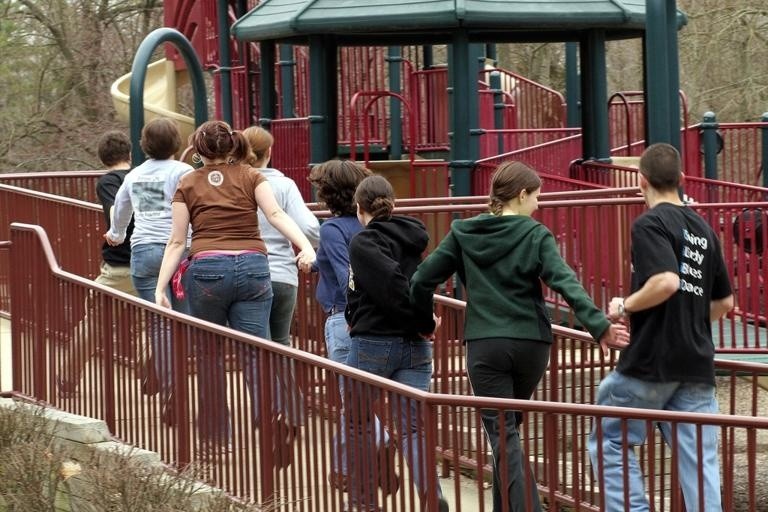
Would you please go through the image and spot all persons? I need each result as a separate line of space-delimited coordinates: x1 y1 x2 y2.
239 127 323 439
102 119 196 428
341 175 450 511
54 129 163 396
296 159 403 495
586 143 732 512
411 161 631 511
156 121 317 469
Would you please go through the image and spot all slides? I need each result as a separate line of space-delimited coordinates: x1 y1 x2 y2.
111 58 195 161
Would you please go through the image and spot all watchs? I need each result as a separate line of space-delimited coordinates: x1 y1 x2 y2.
618 295 632 319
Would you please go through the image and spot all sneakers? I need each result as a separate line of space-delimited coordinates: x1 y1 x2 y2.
195 437 234 456
271 414 294 469
57 373 79 398
143 359 176 425
328 471 350 492
377 446 399 496
